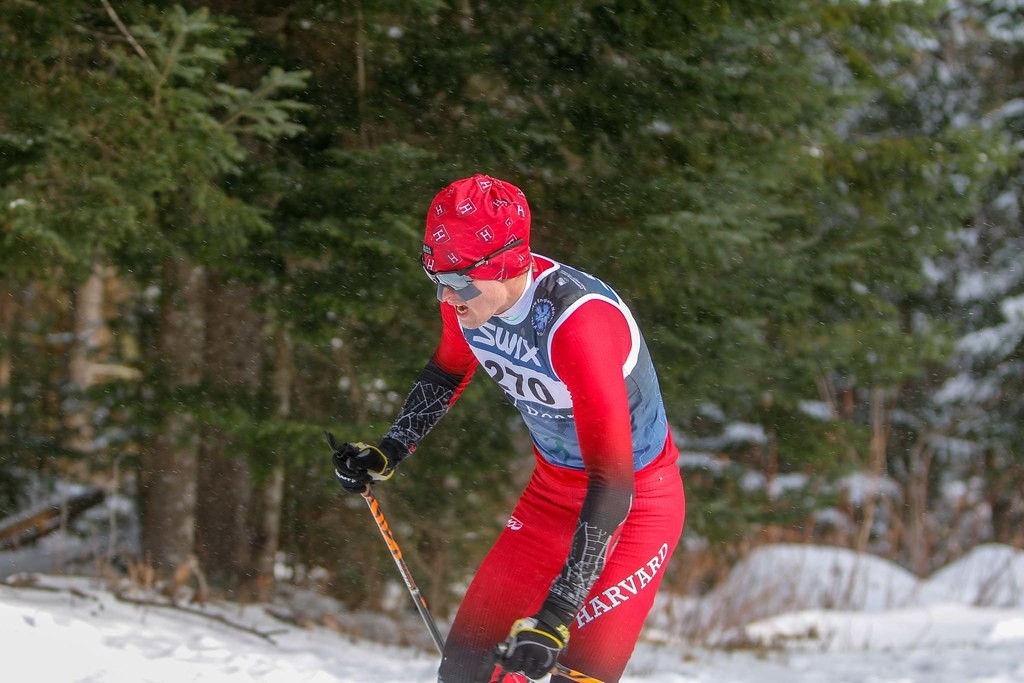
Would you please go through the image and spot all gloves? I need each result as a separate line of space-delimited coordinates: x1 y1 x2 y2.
499 591 581 680
332 435 410 494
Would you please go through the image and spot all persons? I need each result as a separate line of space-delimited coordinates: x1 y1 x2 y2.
332 174 685 683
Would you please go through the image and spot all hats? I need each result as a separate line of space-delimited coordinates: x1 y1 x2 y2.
423 174 531 281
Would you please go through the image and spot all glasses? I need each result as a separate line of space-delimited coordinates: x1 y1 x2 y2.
420 239 522 292
